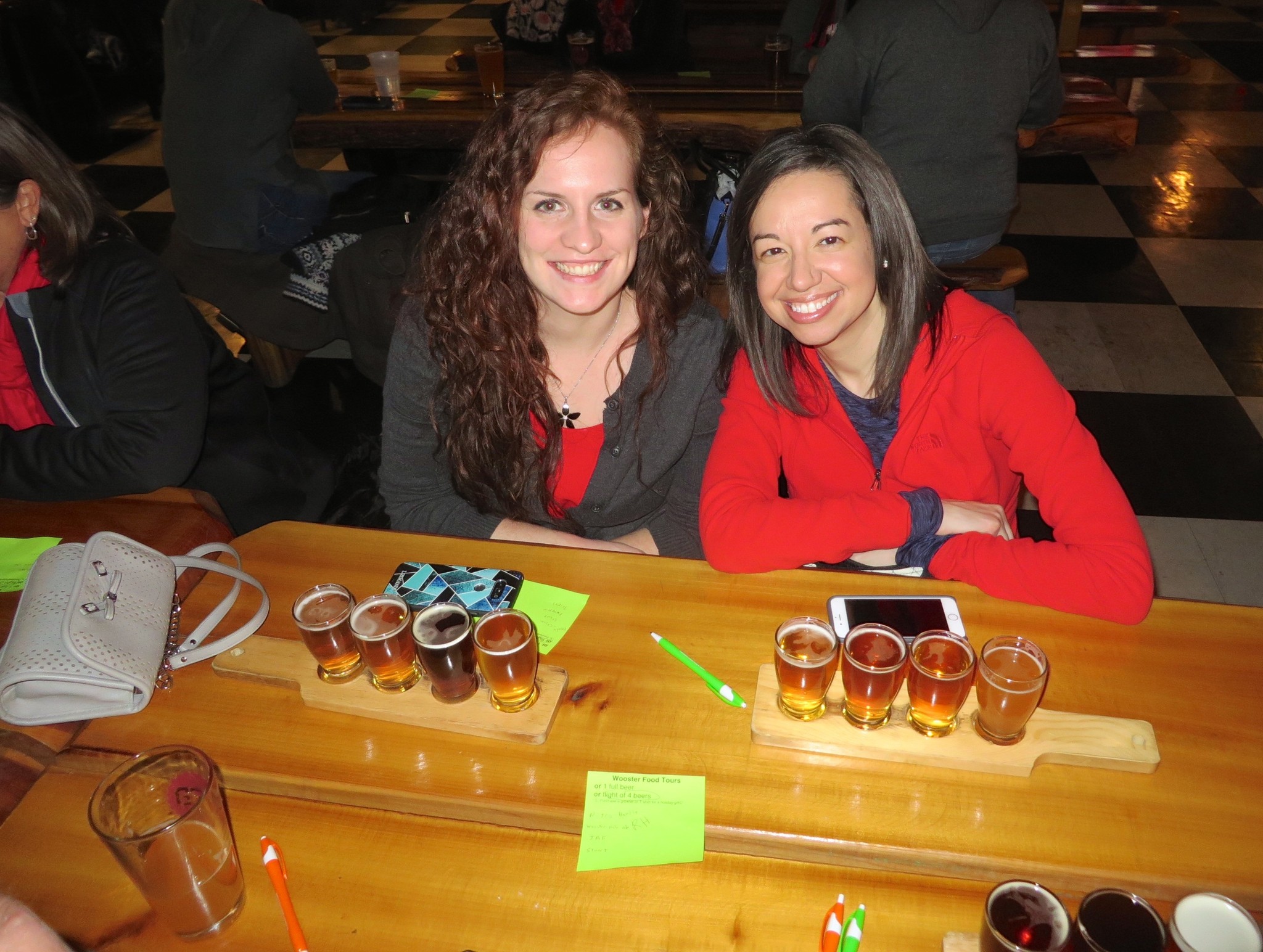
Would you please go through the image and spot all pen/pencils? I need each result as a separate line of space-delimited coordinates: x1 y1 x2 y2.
260 835 309 952
837 904 865 952
650 631 747 708
819 894 844 952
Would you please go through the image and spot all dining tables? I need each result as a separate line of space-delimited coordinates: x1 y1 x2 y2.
0 508 1263 952
285 75 1137 180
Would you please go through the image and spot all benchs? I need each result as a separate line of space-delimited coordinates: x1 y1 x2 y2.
172 247 1029 380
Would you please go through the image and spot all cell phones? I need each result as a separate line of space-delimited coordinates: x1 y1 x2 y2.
383 562 524 617
342 95 392 109
827 595 967 645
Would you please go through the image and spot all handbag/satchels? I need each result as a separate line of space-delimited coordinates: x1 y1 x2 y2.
694 149 740 277
0 530 270 727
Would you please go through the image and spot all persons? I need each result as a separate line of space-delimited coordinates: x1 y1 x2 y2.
697 122 1155 624
380 71 738 562
801 0 1065 298
164 1 337 254
0 103 286 536
775 0 861 130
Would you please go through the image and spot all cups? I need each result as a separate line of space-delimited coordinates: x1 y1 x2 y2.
764 32 792 82
347 593 422 694
292 583 366 683
1073 887 1169 952
321 59 337 83
472 608 539 713
567 30 595 71
1165 891 1262 952
979 879 1072 952
906 630 976 738
366 51 400 96
841 622 908 731
774 616 838 721
87 744 247 940
410 601 479 703
475 42 504 98
976 635 1048 740
